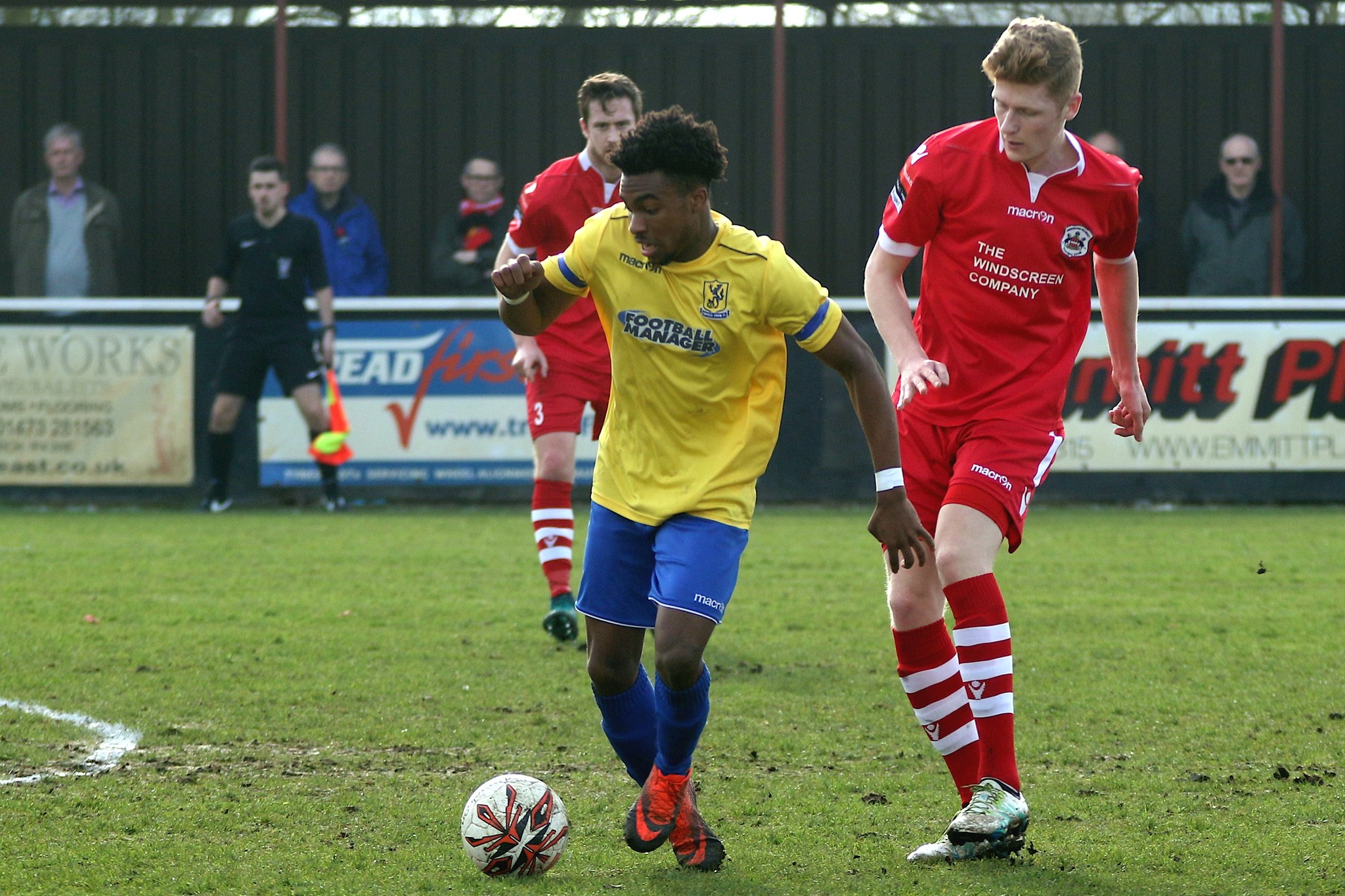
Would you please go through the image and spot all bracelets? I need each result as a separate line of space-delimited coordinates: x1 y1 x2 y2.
502 292 531 306
874 467 907 493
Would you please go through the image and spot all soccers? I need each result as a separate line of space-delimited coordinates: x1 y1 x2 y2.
460 773 569 875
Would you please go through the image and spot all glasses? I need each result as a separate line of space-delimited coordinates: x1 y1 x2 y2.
1222 155 1259 165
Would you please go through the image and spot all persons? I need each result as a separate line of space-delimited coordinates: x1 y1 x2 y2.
1176 133 1308 297
494 69 646 640
202 155 347 511
862 15 1153 865
420 157 514 296
1088 131 1163 299
12 123 136 299
491 104 935 877
287 144 389 296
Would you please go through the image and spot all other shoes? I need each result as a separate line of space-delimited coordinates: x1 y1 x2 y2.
543 592 579 643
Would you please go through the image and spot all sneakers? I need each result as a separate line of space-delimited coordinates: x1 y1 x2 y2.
946 776 1030 846
906 802 1025 864
669 777 733 874
624 760 693 853
